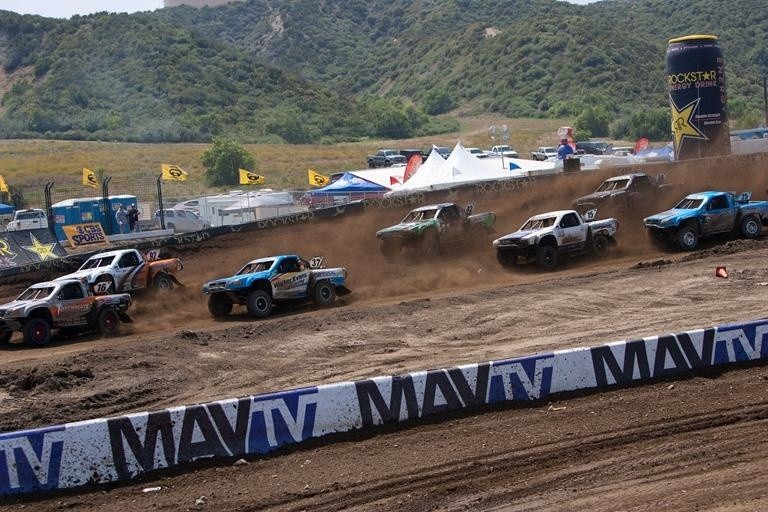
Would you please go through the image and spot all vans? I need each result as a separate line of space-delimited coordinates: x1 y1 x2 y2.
153 208 220 234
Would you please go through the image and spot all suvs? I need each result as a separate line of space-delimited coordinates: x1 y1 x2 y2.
196 253 347 318
0 207 49 234
363 141 636 169
0 275 133 347
51 245 185 296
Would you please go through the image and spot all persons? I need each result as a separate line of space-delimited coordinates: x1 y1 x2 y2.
115 203 129 234
125 203 140 232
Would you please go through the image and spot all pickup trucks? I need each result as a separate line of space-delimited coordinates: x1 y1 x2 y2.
571 172 679 222
491 209 618 270
643 190 767 251
376 203 497 263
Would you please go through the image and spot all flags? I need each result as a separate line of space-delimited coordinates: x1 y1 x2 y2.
0 173 10 193
307 167 330 187
237 167 264 185
159 162 187 182
81 166 100 190
